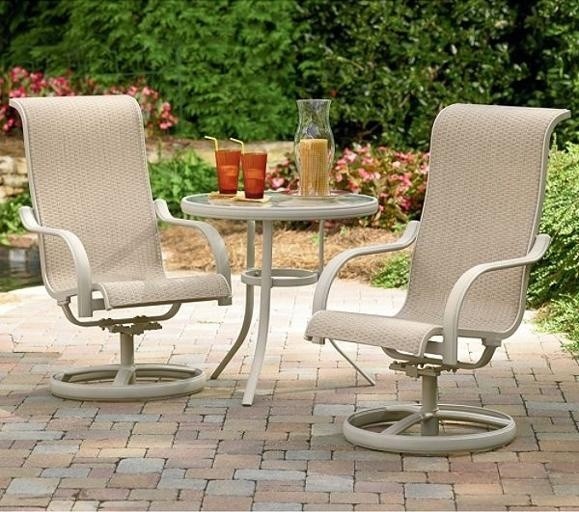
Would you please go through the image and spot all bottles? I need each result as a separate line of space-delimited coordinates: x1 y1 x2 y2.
294 97 336 189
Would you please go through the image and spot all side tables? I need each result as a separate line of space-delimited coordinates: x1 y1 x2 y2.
179 190 379 406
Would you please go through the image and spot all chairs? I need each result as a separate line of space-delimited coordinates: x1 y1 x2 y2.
306 104 573 458
8 93 231 402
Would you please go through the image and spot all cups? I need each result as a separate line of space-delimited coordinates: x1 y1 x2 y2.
214 148 268 198
